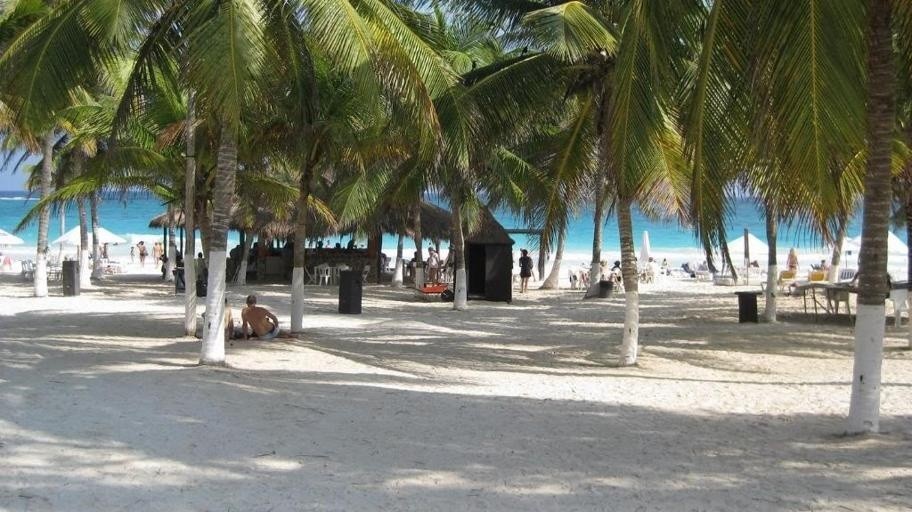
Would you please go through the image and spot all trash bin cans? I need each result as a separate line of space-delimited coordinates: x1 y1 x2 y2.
599 280 613 298
338 270 362 314
63 261 80 296
735 292 762 324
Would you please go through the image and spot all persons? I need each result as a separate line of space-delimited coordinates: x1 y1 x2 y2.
428 246 442 279
129 241 205 284
346 237 357 249
519 249 533 293
568 248 830 290
326 239 334 248
201 297 236 341
240 293 280 339
318 240 323 248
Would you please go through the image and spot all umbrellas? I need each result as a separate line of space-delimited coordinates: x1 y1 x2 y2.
639 230 651 262
69 227 127 245
727 232 772 255
850 229 907 257
54 224 90 257
0 228 24 248
823 234 859 268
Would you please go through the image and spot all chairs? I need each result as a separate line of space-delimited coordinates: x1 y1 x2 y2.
286 248 448 288
567 254 673 297
18 243 124 287
683 254 911 329
